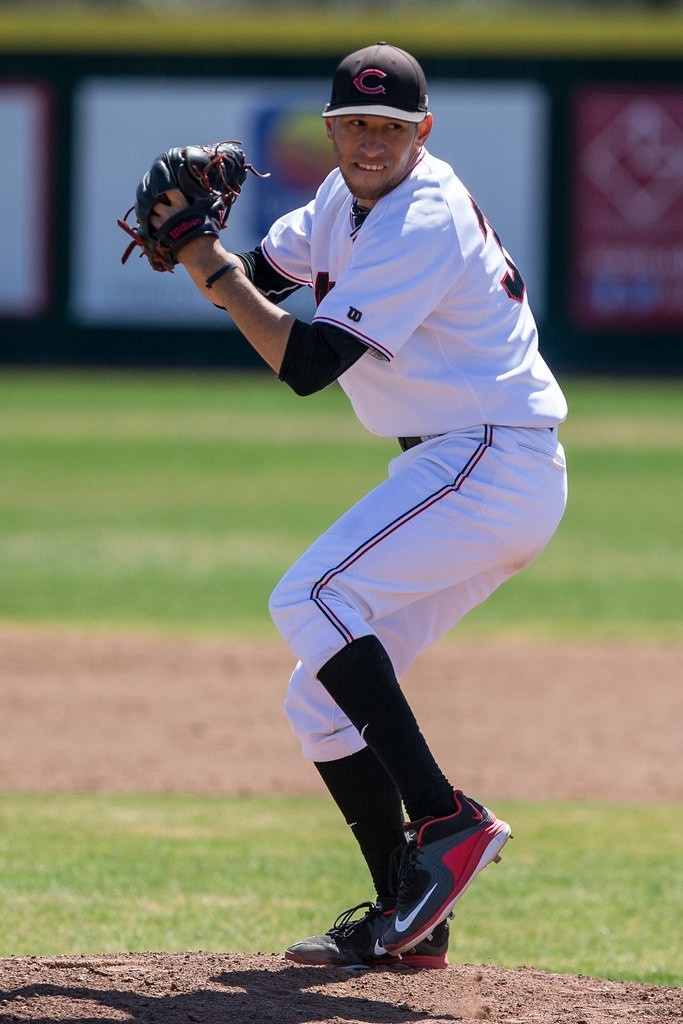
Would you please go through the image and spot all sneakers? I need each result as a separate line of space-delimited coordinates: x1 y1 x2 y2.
284 901 455 970
382 790 514 956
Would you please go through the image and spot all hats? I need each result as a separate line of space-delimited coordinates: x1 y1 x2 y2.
321 42 427 122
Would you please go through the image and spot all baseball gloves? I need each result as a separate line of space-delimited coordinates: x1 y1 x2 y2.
130 140 250 273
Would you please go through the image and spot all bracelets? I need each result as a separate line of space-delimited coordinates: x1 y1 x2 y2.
205 262 238 289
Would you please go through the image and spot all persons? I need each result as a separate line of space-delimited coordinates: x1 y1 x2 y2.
135 42 568 967
